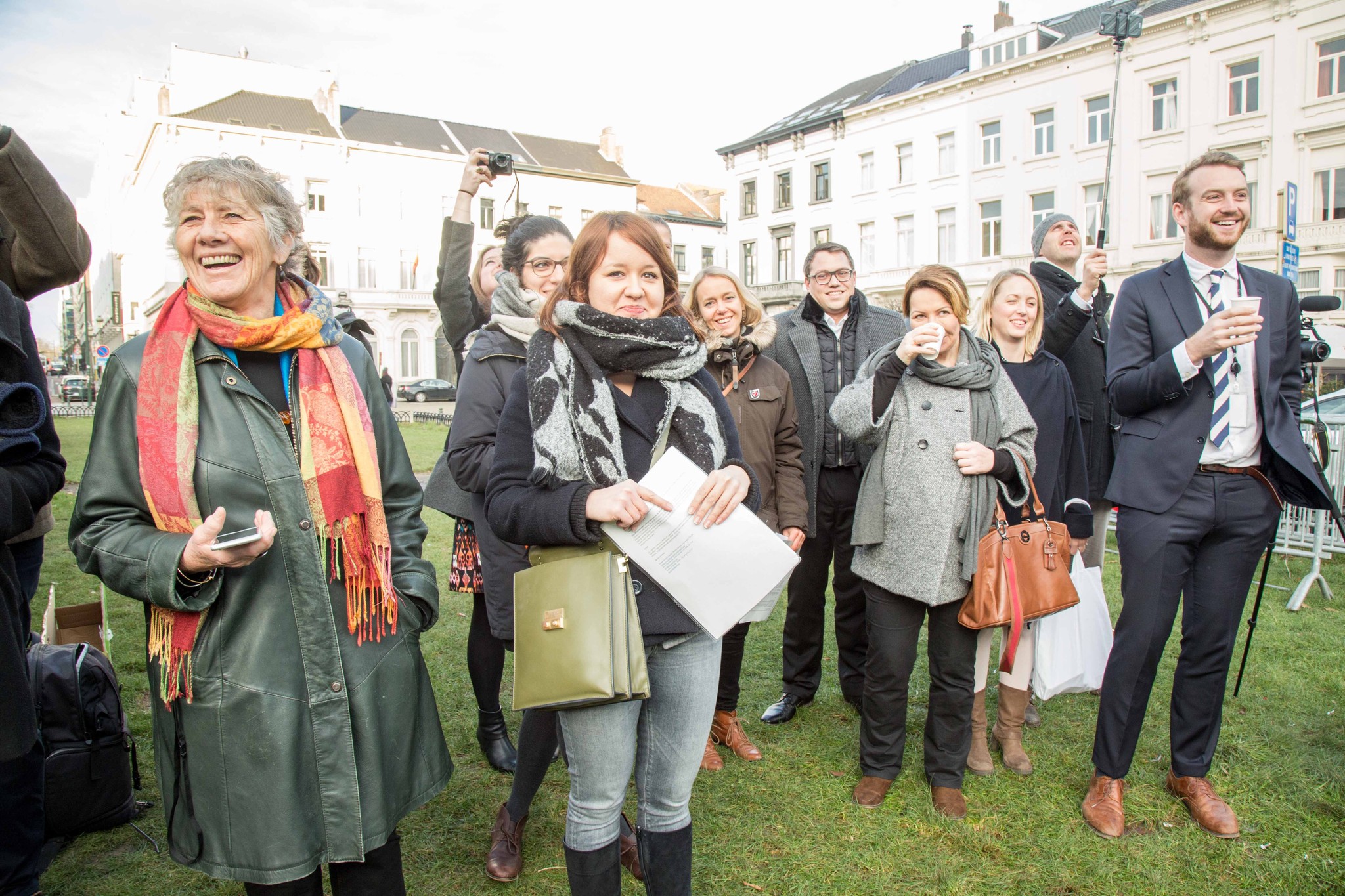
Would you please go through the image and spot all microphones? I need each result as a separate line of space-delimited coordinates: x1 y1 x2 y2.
1296 296 1345 319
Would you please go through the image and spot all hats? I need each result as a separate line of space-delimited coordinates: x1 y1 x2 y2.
1032 214 1077 258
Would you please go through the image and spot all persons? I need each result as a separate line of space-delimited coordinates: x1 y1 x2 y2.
484 210 762 896
67 156 454 896
1023 214 1114 726
679 265 809 771
759 242 911 724
448 214 644 882
966 269 1093 778
1081 152 1345 840
422 147 563 773
0 129 93 896
828 264 1038 820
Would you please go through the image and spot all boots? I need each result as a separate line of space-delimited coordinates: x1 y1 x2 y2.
550 744 561 762
965 686 994 776
476 705 517 772
563 832 621 896
634 821 692 896
991 681 1034 775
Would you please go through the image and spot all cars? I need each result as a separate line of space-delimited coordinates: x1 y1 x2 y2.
397 379 457 403
40 356 69 376
55 375 97 402
1299 388 1345 509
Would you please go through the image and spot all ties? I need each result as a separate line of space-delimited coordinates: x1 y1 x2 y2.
1209 270 1230 449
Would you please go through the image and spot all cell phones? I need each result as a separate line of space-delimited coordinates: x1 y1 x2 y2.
211 526 262 552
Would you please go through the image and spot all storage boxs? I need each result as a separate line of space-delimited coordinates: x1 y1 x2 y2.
40 581 115 667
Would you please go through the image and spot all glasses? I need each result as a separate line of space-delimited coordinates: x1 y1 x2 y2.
517 255 570 276
806 268 855 285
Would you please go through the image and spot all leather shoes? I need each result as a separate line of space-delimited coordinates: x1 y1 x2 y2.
852 774 893 807
486 802 530 882
712 710 763 761
1164 765 1239 838
620 812 644 882
1089 688 1100 696
1080 767 1129 838
854 700 863 717
700 725 724 770
932 786 967 820
1024 703 1040 727
761 692 814 725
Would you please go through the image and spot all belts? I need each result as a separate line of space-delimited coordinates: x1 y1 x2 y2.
1198 464 1283 511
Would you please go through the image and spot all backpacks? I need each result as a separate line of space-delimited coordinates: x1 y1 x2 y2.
26 632 141 833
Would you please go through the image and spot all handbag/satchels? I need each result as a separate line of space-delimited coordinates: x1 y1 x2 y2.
1032 548 1114 700
956 447 1080 629
513 535 650 712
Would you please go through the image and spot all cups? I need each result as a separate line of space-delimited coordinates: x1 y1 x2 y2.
921 322 945 361
1229 296 1262 336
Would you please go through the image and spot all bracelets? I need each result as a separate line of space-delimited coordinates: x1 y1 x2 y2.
459 189 474 197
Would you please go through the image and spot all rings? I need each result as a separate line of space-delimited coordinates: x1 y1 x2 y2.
255 550 268 559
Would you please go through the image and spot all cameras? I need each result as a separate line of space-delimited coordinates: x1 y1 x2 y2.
478 151 512 176
